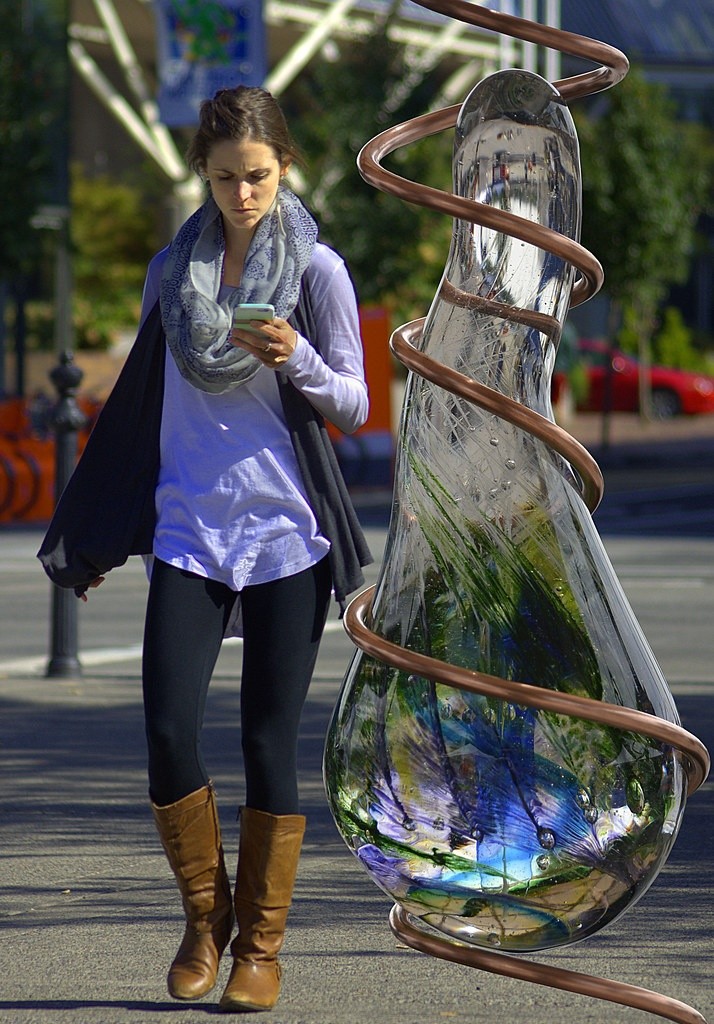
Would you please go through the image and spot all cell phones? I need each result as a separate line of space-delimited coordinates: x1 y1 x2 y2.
234 304 274 343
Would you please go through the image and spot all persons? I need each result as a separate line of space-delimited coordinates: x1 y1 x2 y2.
35 86 375 1013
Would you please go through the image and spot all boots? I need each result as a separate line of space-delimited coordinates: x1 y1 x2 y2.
149 777 234 1002
219 806 308 1009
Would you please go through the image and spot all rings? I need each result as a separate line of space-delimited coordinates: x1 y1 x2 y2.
263 341 272 353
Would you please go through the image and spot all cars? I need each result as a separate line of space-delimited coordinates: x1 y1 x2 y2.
549 336 714 420
